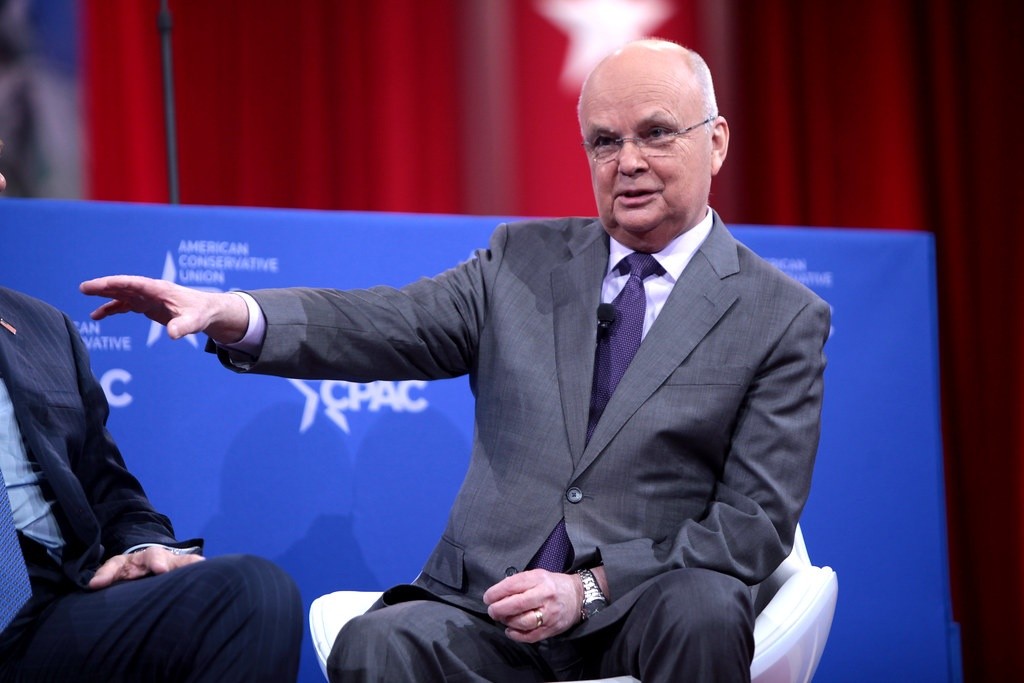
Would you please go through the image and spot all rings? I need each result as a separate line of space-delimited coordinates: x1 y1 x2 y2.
532 609 544 627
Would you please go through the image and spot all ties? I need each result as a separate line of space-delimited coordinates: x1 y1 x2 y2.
0 468 33 633
527 252 660 573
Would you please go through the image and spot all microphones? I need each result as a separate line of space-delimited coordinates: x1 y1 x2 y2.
597 303 614 329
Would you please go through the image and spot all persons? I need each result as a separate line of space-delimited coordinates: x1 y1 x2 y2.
80 36 835 682
0 163 308 683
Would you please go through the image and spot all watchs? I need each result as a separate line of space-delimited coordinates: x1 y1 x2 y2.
577 562 609 620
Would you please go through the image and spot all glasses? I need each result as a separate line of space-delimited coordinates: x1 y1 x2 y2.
581 116 716 165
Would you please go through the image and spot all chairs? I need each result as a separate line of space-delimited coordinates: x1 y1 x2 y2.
309 521 839 683
